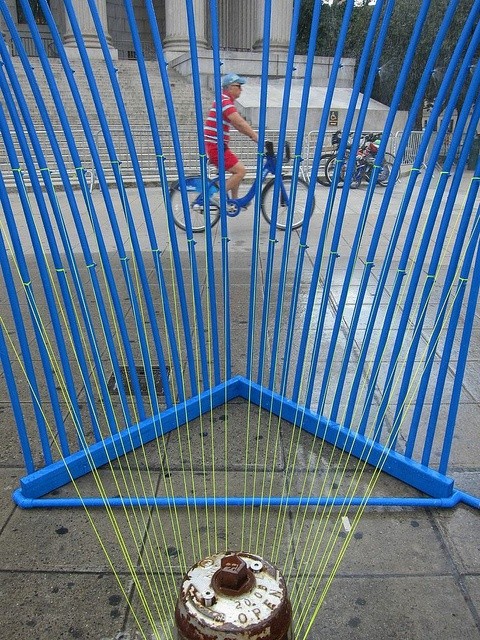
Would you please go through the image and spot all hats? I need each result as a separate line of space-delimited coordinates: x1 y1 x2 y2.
223 74 246 86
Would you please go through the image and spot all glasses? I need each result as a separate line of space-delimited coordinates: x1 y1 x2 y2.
231 84 241 89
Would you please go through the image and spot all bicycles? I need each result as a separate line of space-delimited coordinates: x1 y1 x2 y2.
169 140 316 233
317 131 401 189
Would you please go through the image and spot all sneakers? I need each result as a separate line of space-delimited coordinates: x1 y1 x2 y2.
209 192 233 211
244 201 253 209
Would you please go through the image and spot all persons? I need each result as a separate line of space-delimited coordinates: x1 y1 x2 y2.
188 72 272 214
